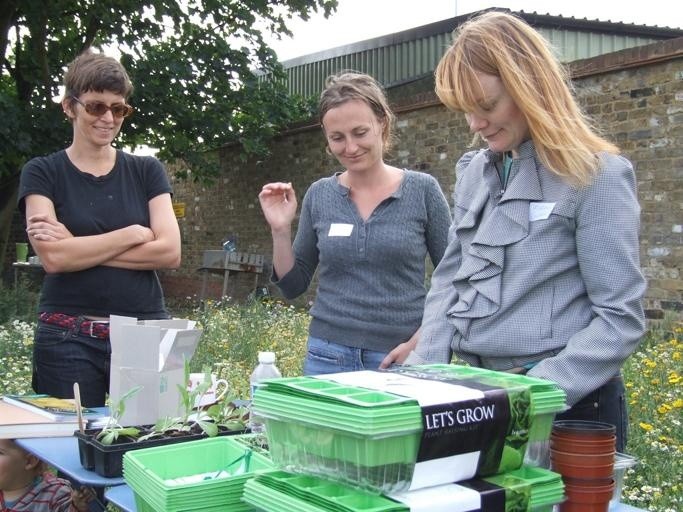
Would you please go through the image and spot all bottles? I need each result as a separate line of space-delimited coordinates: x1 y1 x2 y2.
248 350 284 437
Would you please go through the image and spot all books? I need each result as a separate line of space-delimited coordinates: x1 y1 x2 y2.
0 389 107 439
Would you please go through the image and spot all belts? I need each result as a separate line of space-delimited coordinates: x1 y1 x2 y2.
37 310 111 341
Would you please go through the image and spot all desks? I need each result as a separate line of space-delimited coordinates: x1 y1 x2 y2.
13 406 127 486
103 486 648 512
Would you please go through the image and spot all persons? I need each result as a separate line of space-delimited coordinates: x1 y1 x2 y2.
256 69 454 378
18 52 181 506
0 439 96 512
404 13 647 453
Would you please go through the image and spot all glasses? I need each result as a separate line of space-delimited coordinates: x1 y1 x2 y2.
73 95 132 119
